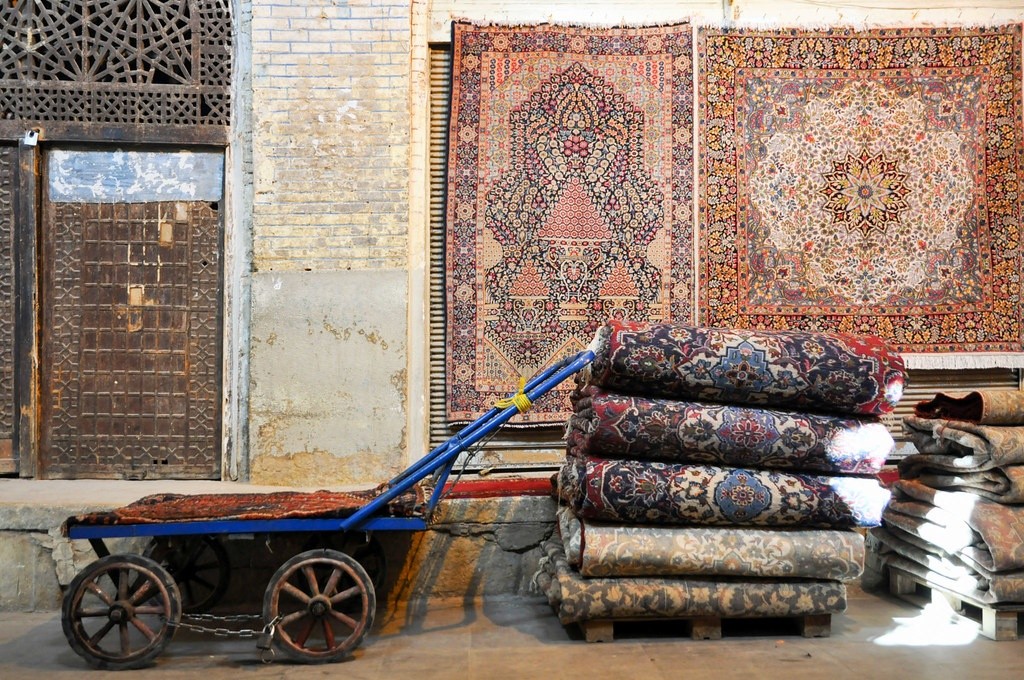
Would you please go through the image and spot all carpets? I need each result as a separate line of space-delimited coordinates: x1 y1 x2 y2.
60 480 441 539
445 16 1024 624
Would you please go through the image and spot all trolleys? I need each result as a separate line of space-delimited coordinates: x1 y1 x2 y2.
55 349 595 672
